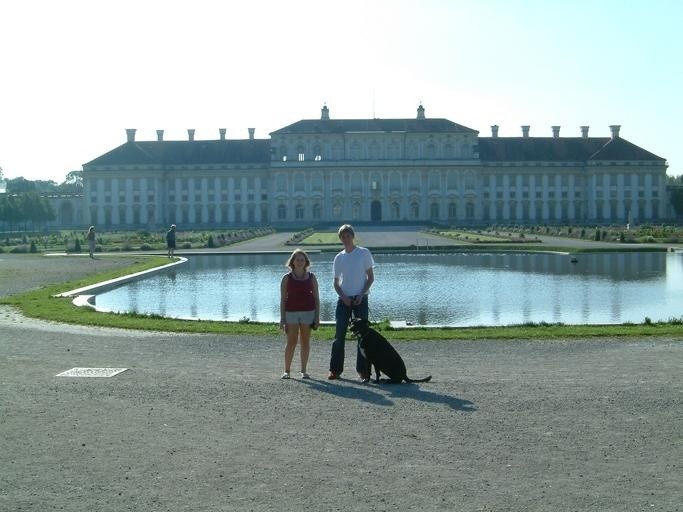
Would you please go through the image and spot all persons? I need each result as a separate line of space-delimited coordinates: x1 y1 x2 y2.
166 224 176 258
86 226 95 258
279 249 319 379
327 224 375 379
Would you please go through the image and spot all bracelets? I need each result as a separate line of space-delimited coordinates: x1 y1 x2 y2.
280 320 287 323
313 320 319 324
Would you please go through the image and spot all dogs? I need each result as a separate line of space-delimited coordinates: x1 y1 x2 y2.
347 317 433 384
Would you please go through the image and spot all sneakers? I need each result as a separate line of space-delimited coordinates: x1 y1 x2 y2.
360 373 369 381
301 373 308 378
328 372 340 379
281 373 289 379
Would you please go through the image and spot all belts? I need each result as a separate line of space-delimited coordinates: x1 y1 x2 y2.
339 295 368 299
